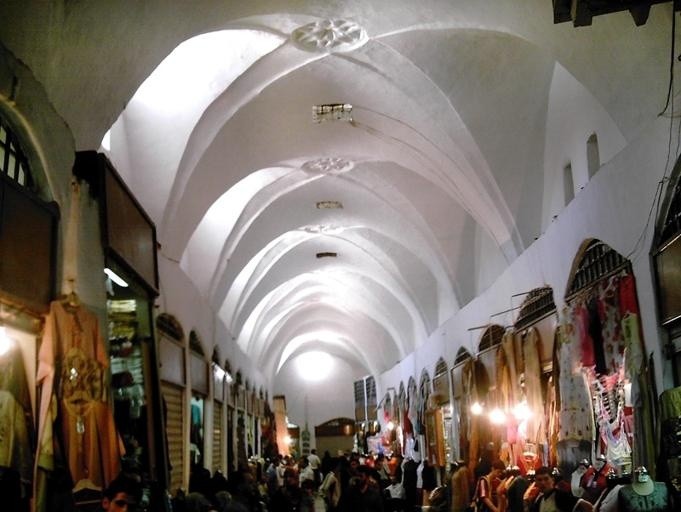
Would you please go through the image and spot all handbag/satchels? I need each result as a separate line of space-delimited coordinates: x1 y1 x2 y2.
470 497 480 512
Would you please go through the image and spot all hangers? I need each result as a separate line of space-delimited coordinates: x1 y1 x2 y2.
563 269 626 312
64 373 94 415
58 275 84 314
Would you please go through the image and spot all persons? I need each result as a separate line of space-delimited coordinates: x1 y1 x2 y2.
593 456 668 512
103 448 593 512
